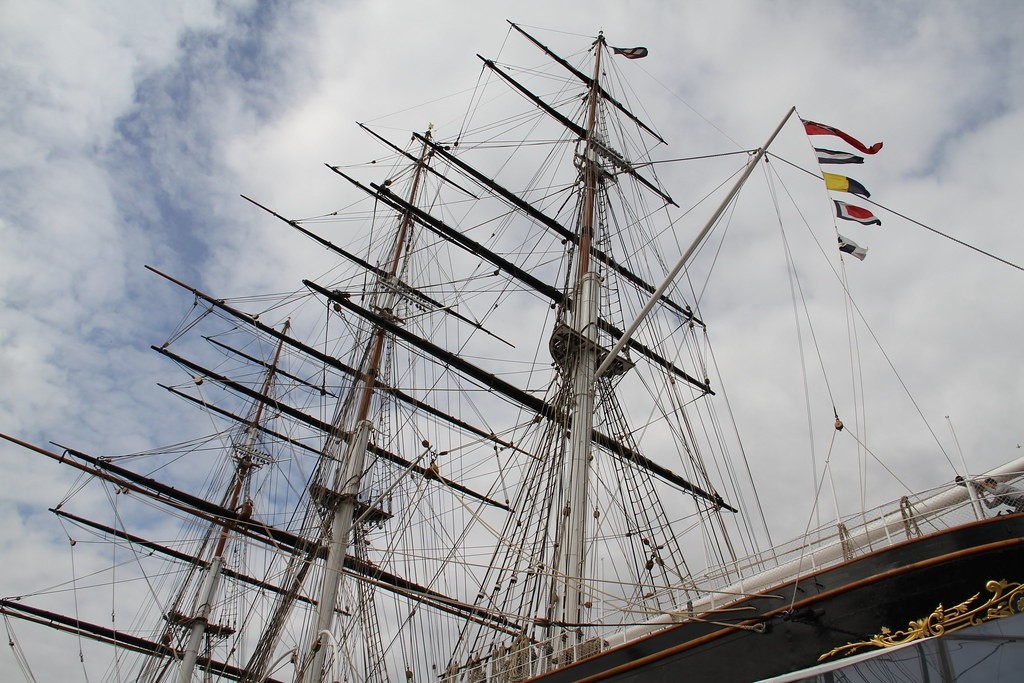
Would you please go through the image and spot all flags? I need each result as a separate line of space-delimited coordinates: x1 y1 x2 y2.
837 232 868 261
799 117 884 154
814 147 865 164
822 170 872 198
832 199 881 226
